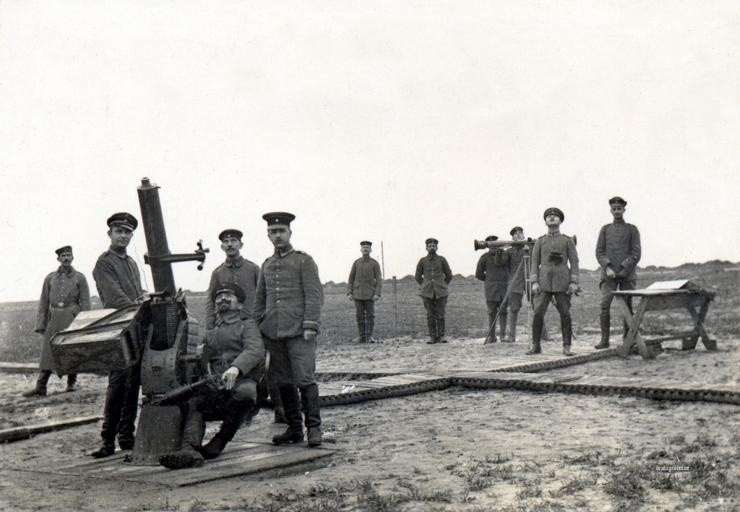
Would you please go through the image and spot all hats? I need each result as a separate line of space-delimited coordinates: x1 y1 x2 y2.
262 211 296 226
425 238 438 244
211 281 245 303
543 207 564 223
54 246 72 255
510 227 523 235
360 241 372 246
219 229 244 240
609 197 627 206
105 212 138 232
485 236 498 242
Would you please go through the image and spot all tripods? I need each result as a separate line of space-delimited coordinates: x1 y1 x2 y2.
484 255 577 351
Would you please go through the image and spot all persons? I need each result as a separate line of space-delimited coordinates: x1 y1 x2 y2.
21 245 89 398
90 212 144 461
413 239 453 345
474 207 581 356
346 241 384 343
595 195 641 351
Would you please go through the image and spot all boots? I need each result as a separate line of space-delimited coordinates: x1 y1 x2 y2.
300 382 323 446
365 318 377 343
594 312 610 349
525 316 542 355
66 372 78 392
157 410 206 469
560 314 577 355
352 321 365 342
23 369 55 396
485 316 497 344
272 383 304 443
118 388 139 448
499 311 517 342
621 313 634 343
436 318 448 343
426 320 438 344
202 400 251 459
533 318 553 343
92 385 124 457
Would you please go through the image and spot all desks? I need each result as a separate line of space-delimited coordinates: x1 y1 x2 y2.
610 284 719 361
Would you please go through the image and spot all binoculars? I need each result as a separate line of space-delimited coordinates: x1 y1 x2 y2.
123 177 210 466
474 234 579 251
